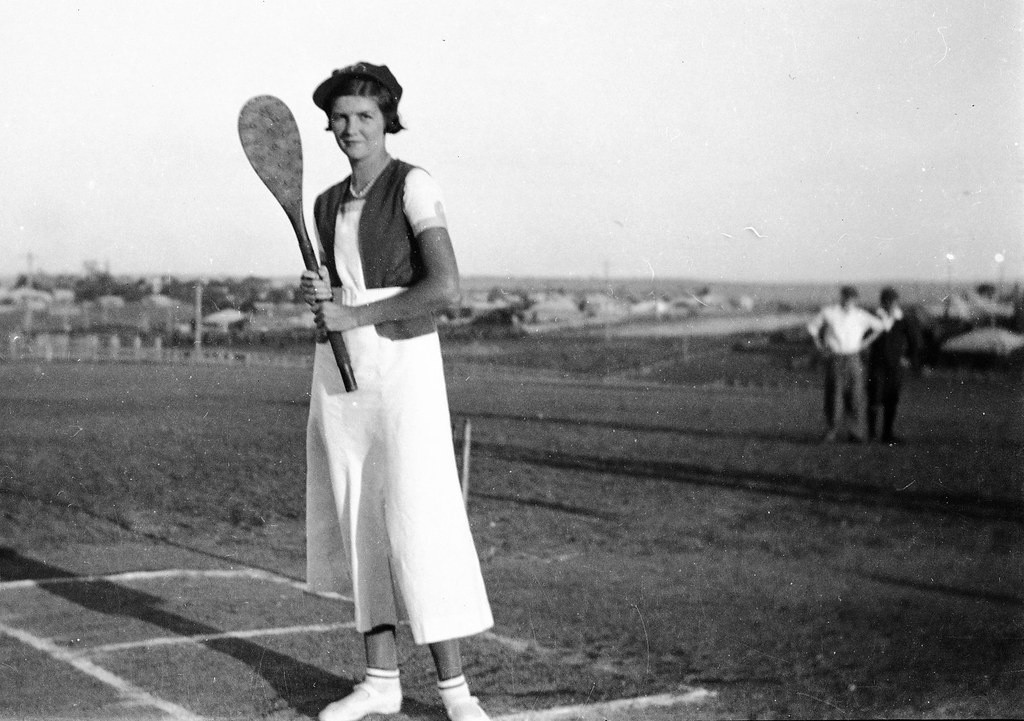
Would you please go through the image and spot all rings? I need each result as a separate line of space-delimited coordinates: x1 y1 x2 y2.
314 287 317 294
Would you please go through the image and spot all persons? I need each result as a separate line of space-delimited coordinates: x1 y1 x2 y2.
298 62 495 720
865 288 909 445
807 286 885 442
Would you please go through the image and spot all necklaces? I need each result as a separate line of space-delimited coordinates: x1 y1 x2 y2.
349 178 376 198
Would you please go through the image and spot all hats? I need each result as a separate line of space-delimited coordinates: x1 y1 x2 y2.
312 63 403 113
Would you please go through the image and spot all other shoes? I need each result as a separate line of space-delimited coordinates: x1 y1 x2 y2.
319 681 405 721
446 694 491 721
